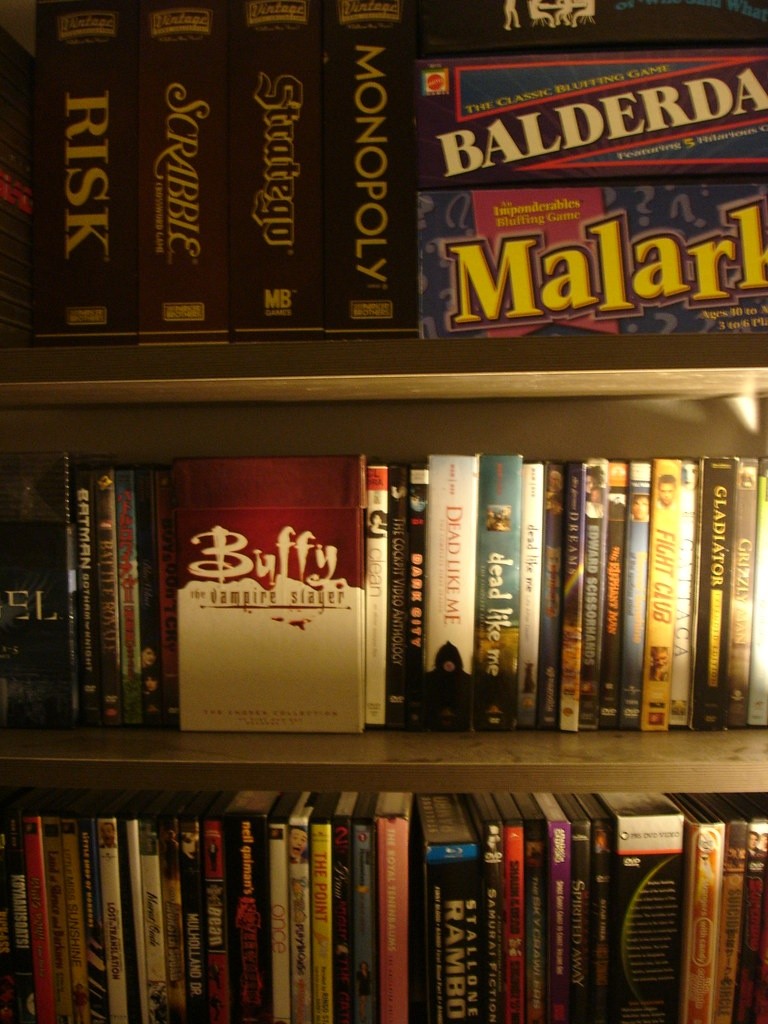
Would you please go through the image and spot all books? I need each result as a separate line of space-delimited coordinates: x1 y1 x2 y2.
7 787 768 1024
1 452 767 732
0 1 768 335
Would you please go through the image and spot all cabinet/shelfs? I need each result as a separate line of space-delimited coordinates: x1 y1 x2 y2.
0 332 768 786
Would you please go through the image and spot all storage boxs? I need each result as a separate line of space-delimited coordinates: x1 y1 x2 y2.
410 0 768 340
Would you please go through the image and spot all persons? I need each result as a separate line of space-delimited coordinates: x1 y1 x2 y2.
681 463 698 489
288 827 307 864
73 984 89 1024
583 464 605 520
656 474 677 509
141 644 160 712
648 647 669 681
357 959 371 1023
629 494 650 523
98 821 117 848
180 832 201 870
